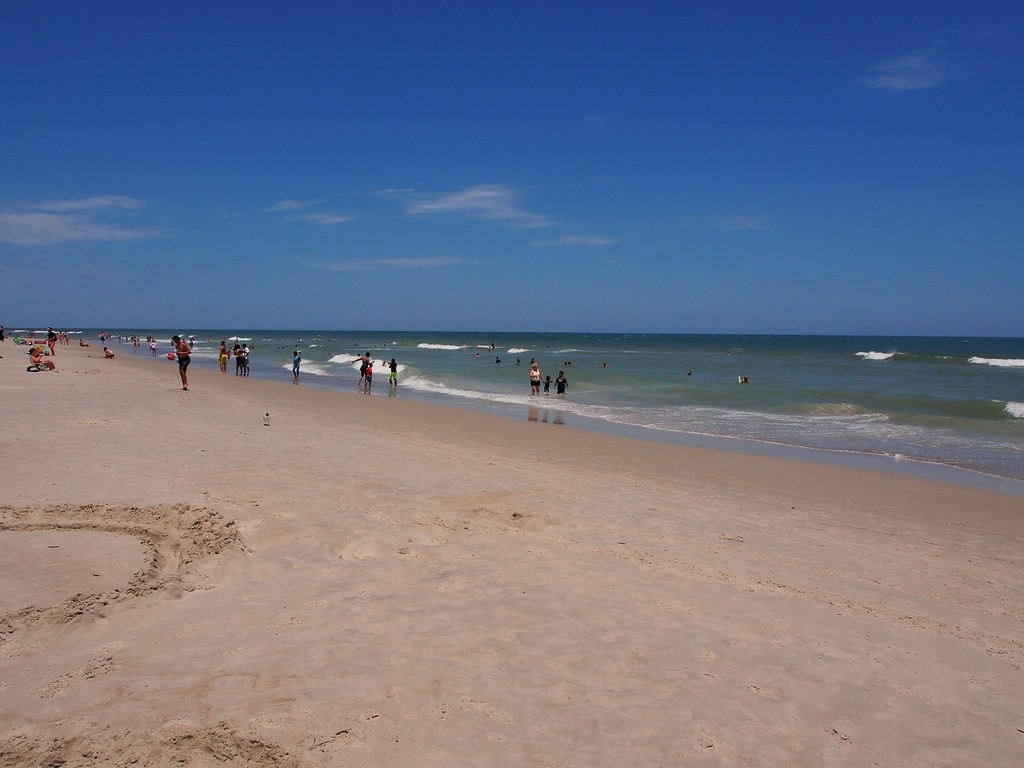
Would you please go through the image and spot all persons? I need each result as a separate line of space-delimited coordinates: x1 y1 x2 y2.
0 325 749 419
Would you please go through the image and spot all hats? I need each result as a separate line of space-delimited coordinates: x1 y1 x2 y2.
532 363 538 368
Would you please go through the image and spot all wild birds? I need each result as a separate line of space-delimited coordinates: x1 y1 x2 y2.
263 412 271 426
